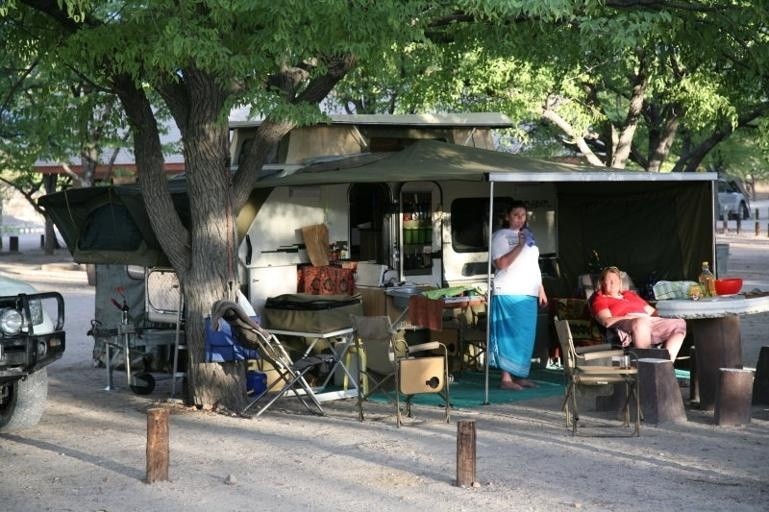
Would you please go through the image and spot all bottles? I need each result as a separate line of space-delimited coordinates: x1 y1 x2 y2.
699 262 715 298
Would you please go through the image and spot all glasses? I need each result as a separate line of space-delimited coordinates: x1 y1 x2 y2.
602 266 619 272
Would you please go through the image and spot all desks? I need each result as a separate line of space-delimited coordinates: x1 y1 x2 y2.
261 327 362 403
654 283 769 410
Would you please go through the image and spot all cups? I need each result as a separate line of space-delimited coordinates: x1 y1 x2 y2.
520 225 535 247
689 285 702 300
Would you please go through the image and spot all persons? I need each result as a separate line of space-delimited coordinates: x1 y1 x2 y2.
592 266 687 363
490 201 547 390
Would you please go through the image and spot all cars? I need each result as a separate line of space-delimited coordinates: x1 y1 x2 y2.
718 179 747 220
0 275 65 433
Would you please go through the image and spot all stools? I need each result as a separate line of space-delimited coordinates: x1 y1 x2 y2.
632 346 769 427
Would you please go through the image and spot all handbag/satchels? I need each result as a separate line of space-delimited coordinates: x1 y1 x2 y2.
653 280 704 301
203 314 261 363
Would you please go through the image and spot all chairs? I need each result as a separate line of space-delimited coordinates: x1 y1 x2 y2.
577 271 640 342
349 312 421 425
553 314 648 435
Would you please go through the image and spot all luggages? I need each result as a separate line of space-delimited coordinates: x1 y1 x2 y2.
264 294 362 333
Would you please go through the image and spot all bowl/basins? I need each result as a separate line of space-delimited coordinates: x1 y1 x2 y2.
716 279 743 295
